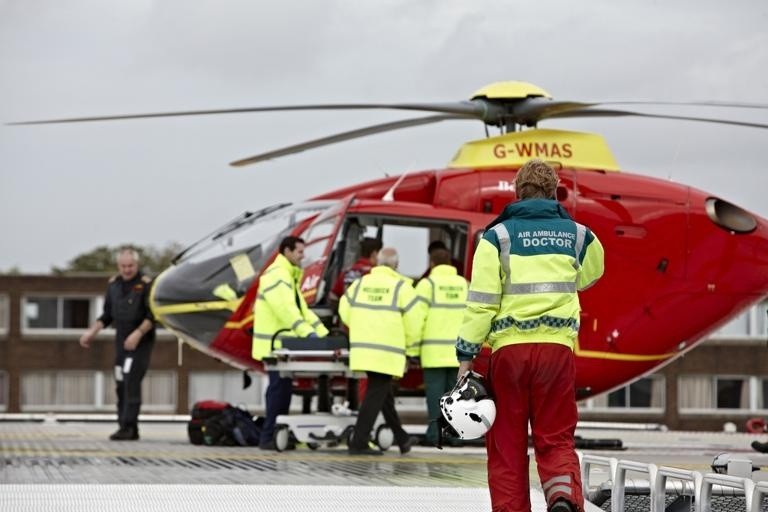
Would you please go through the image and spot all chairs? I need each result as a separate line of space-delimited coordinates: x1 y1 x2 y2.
312 214 364 329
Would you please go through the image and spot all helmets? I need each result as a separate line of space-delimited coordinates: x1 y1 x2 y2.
438 370 498 440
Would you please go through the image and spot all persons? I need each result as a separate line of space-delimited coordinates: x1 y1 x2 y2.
325 236 384 335
249 235 331 453
418 240 464 278
337 246 419 456
77 245 160 440
411 247 473 449
451 157 607 510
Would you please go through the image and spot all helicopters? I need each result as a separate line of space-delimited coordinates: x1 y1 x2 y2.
4 80 768 451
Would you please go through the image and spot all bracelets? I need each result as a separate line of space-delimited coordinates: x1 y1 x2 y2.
138 326 146 338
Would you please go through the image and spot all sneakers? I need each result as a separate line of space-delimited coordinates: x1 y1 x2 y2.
258 439 296 451
349 442 385 455
400 435 416 454
110 427 140 441
